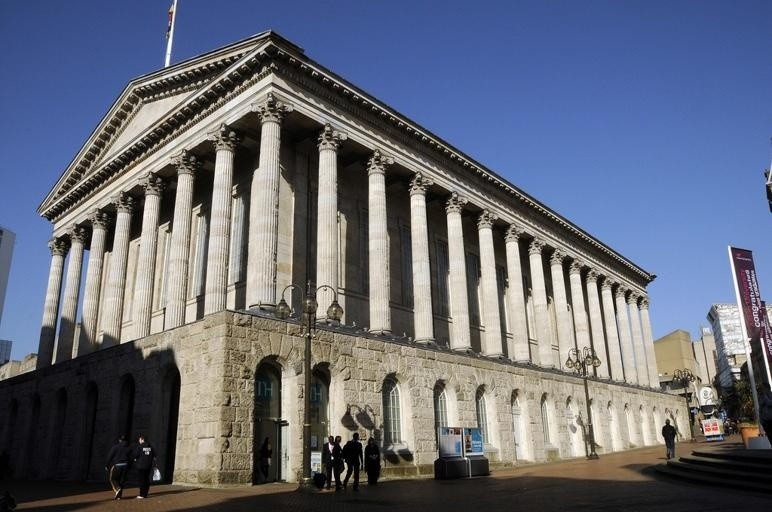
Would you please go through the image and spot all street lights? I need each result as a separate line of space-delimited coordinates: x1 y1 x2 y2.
671 368 697 443
565 346 602 460
275 279 344 492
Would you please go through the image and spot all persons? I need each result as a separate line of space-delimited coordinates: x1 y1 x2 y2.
321 435 334 490
365 435 381 486
722 418 740 436
333 435 345 489
259 435 273 482
661 418 677 459
129 434 156 499
104 435 132 499
341 432 364 492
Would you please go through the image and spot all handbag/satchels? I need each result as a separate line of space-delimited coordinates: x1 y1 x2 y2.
152 466 162 481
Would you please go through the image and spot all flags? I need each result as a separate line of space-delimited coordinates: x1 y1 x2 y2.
166 4 174 40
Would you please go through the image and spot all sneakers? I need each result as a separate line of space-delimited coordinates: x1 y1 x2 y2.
137 495 144 499
115 488 122 500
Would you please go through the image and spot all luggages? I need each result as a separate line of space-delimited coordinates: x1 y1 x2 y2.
312 465 327 490
